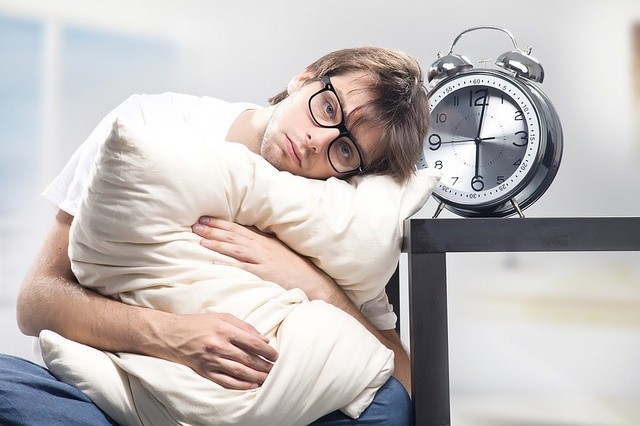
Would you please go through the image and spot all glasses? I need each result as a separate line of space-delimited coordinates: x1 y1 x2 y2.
308 74 366 176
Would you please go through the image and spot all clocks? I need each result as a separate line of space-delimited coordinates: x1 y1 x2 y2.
415 24 563 219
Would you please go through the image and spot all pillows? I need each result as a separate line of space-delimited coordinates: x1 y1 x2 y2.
38 117 444 425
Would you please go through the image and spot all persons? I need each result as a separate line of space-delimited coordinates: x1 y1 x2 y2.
0 46 430 426
